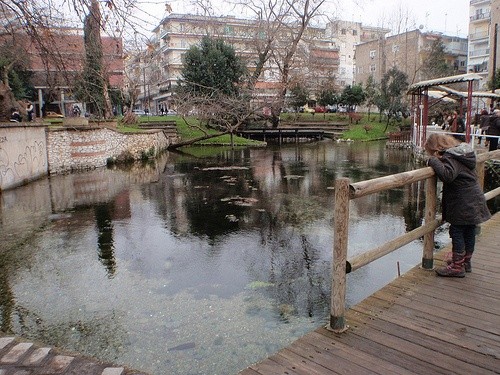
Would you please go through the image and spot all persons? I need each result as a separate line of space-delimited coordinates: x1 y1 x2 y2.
9 101 169 122
435 106 500 152
416 134 492 279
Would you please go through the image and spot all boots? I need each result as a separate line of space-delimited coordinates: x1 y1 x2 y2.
435 251 466 278
446 253 472 273
